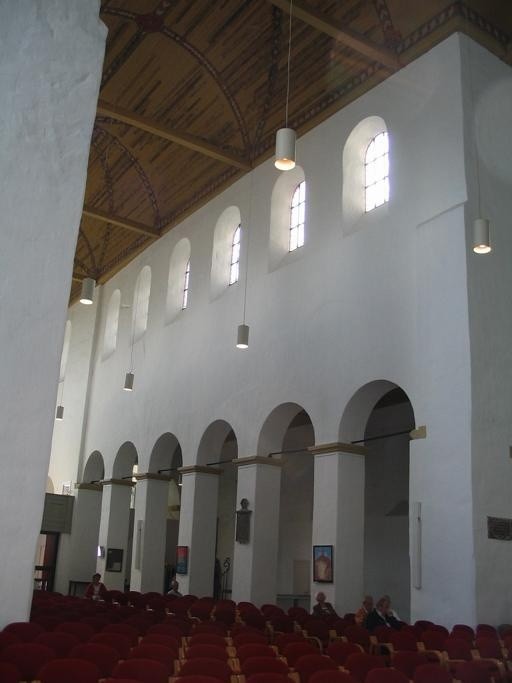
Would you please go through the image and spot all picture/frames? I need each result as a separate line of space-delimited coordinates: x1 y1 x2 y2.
175 545 188 574
313 545 333 583
106 548 123 572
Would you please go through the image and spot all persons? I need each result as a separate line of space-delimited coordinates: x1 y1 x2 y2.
354 595 373 627
83 572 107 600
167 579 183 613
380 594 402 622
364 595 400 634
312 591 339 618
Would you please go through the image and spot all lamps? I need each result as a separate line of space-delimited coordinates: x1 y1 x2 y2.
79 85 125 305
56 298 71 420
237 147 259 349
124 238 142 391
472 1 492 254
274 1 296 171
